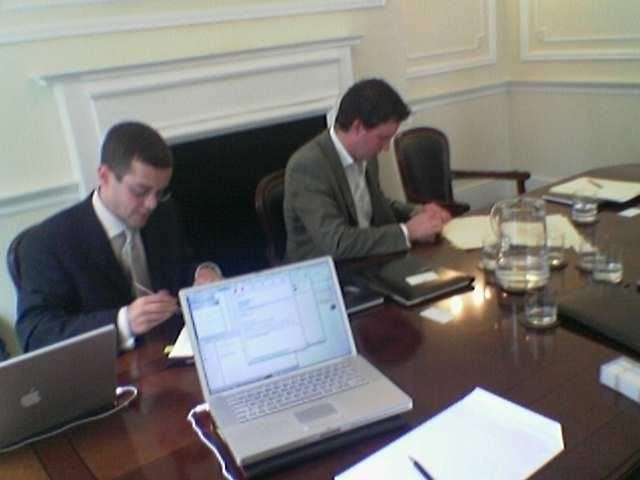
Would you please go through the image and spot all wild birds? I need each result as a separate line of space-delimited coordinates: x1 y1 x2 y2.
0 323 118 452
178 254 414 467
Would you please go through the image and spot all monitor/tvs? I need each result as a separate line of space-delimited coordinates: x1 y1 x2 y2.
342 255 476 306
337 272 386 315
541 177 640 211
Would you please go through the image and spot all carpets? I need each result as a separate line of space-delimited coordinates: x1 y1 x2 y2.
121 228 153 298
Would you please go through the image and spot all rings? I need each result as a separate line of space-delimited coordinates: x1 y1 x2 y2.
134 281 154 295
409 456 433 480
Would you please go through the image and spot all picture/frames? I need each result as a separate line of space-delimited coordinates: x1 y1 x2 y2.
0 161 639 479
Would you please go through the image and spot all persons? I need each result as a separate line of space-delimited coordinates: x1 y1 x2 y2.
284 78 454 266
14 123 227 359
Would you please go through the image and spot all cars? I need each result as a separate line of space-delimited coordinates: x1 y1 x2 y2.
480 233 511 272
539 189 624 285
523 277 560 325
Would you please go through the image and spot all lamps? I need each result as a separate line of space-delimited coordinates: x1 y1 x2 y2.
5 225 25 287
392 125 531 223
255 169 285 269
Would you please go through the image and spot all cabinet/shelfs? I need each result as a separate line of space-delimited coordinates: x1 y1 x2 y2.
489 197 550 293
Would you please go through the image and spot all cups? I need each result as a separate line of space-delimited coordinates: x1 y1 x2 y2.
119 178 172 203
